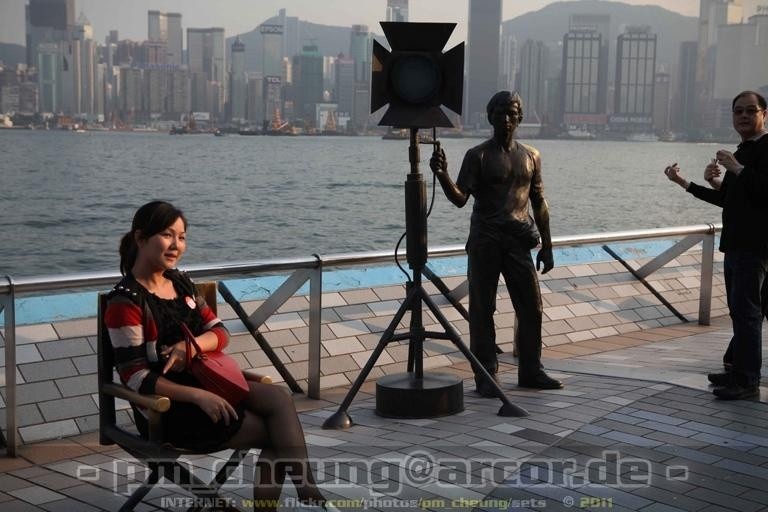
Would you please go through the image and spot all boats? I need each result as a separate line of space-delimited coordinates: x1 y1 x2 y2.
382 130 440 144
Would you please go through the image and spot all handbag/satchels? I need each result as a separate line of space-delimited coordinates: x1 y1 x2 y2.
177 319 250 404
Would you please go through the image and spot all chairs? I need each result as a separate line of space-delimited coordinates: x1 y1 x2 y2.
98 281 271 510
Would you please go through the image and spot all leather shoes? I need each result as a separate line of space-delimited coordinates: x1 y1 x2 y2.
707 369 760 400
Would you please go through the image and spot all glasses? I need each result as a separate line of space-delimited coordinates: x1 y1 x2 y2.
731 107 764 117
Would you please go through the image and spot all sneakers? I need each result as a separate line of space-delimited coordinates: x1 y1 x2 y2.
294 496 342 512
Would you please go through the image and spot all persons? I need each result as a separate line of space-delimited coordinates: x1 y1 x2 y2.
429 89 565 397
103 199 340 511
703 157 767 323
662 92 767 402
